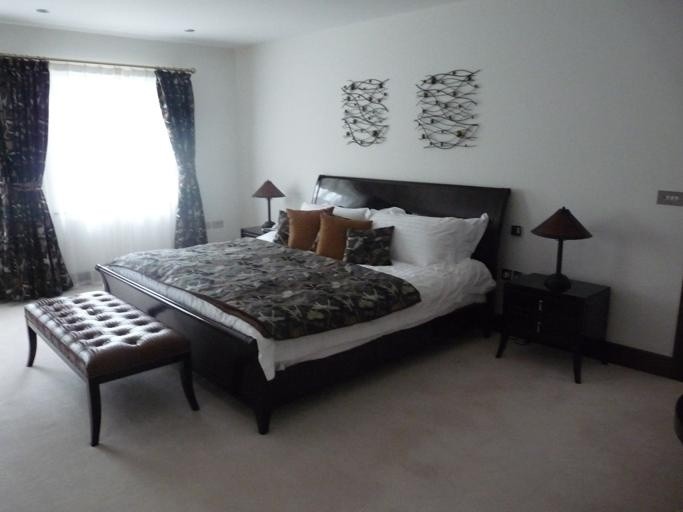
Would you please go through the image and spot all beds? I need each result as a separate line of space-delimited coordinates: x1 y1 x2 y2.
94 174 512 435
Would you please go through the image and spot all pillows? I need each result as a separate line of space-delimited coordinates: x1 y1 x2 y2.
273 200 489 271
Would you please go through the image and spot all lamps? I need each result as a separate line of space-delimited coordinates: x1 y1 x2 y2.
531 206 593 292
250 180 287 230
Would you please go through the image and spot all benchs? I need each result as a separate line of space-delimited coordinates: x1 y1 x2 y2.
21 288 200 446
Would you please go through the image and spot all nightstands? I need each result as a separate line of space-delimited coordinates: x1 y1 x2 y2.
240 225 268 239
494 272 612 385
